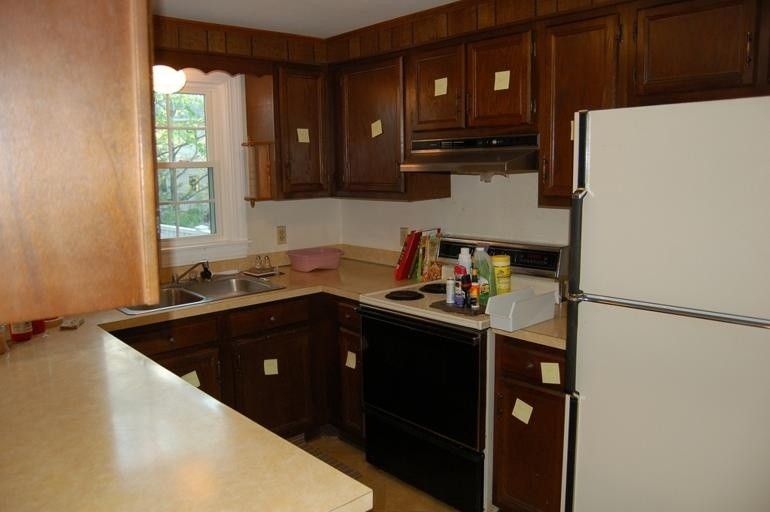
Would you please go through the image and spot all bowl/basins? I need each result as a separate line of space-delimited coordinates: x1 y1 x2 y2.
283 246 346 272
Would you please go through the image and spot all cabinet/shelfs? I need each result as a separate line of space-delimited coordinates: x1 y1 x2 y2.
488 329 566 512
117 292 329 440
335 56 452 202
152 12 328 63
403 23 536 140
245 64 334 200
631 2 770 105
538 7 618 209
327 293 366 434
329 1 616 67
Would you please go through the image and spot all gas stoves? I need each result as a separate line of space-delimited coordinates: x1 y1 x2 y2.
358 306 492 511
359 267 556 330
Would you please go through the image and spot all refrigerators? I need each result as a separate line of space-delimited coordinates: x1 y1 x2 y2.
558 94 770 512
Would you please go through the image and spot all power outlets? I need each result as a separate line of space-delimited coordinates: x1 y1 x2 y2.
277 225 288 245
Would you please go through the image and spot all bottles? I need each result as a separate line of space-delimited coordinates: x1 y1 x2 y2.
454 266 465 306
458 247 472 274
462 272 471 308
446 277 455 306
473 248 494 304
470 277 480 309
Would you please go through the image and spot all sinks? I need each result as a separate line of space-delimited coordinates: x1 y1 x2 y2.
186 274 287 301
116 287 206 316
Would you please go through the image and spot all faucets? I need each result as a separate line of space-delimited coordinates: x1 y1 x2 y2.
171 259 209 286
